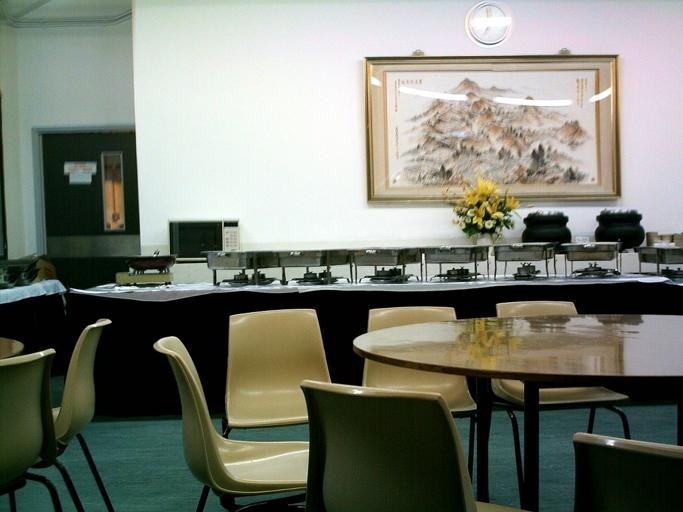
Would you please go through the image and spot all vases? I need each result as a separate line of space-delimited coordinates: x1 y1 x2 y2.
476 232 500 274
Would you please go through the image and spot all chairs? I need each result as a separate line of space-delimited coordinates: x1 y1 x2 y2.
300 380 527 512
364 305 476 485
35 317 115 511
222 308 332 439
574 430 683 511
493 300 631 512
0 349 65 512
155 335 310 512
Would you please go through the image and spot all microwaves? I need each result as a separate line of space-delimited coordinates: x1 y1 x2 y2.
168 218 241 262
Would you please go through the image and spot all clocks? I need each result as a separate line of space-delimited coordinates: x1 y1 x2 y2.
467 1 509 44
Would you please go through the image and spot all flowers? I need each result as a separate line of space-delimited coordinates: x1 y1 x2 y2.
442 175 518 237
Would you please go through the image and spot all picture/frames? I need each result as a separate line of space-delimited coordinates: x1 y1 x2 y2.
361 54 622 203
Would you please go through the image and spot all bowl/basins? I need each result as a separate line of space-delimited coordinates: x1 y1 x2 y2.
278 249 353 267
493 241 559 262
561 241 622 262
200 249 279 270
353 246 423 266
634 245 683 265
424 245 490 264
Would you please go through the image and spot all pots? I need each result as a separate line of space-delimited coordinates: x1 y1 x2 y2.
127 251 176 269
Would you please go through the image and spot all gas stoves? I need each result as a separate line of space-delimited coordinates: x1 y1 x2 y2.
115 268 174 287
